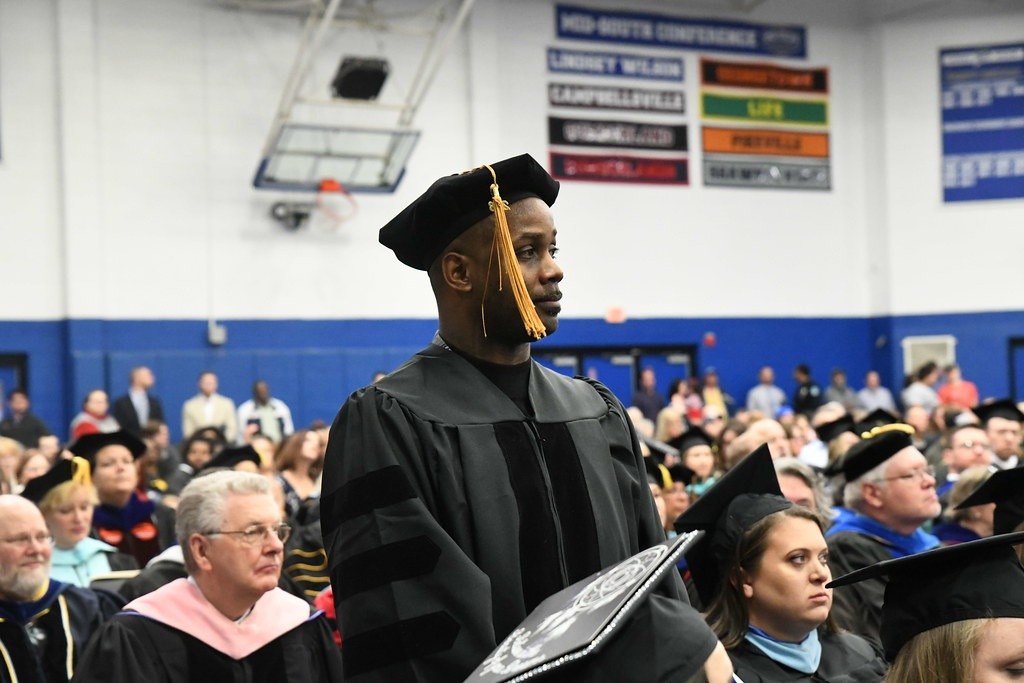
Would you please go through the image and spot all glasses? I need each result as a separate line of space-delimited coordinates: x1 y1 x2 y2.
200 523 292 544
952 438 991 448
876 464 936 483
1 529 56 551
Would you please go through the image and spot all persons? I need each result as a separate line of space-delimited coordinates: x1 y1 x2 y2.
1 360 1024 683
319 154 690 683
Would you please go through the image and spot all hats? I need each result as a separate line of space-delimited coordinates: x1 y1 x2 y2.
953 466 1024 536
203 444 261 468
812 408 915 483
18 455 94 506
673 443 797 608
701 404 725 420
640 425 717 487
69 428 147 475
825 532 1024 661
379 153 560 340
971 398 1024 423
918 360 936 380
463 530 717 683
